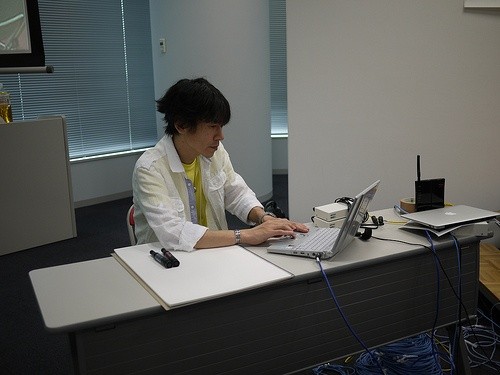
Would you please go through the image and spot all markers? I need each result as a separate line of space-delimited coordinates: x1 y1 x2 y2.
149 247 180 269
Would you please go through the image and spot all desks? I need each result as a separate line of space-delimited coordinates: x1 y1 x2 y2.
28 208 494 375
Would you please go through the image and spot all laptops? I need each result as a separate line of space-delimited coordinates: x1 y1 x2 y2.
399 204 500 230
267 179 380 259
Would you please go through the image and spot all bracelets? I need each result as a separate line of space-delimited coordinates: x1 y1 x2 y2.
234 229 241 244
259 212 277 224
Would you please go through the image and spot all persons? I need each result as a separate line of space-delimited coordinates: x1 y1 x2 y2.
132 77 309 253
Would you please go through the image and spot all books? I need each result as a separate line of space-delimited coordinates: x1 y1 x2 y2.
111 241 295 310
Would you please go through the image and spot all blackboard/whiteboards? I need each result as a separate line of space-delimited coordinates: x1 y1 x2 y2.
0 115 77 258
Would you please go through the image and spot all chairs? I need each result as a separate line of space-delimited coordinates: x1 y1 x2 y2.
126 204 136 246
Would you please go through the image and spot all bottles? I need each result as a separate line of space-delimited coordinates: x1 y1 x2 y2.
0 83 13 123
